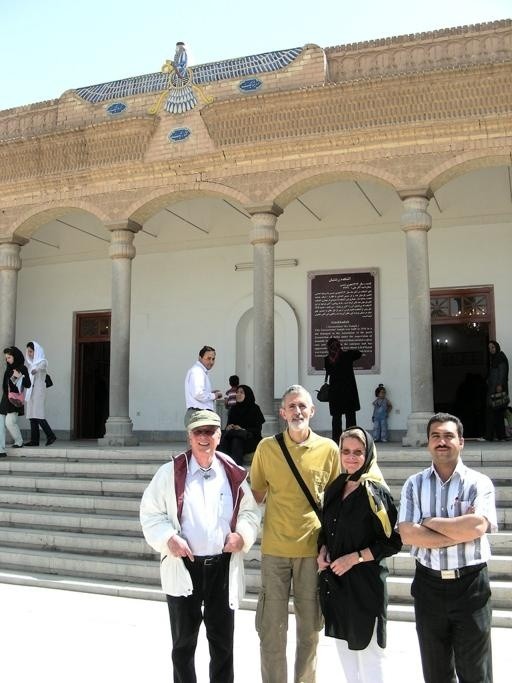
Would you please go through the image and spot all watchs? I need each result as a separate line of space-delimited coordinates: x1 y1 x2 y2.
418 517 426 525
357 550 363 563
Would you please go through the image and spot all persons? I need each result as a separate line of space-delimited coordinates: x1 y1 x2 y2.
476 340 508 442
316 426 402 683
394 412 498 683
249 385 342 683
324 338 362 446
23 341 56 446
139 410 261 683
216 385 264 466
184 346 222 426
0 346 31 458
372 384 393 442
225 375 239 409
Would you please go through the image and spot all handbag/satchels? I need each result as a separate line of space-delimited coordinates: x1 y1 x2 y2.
7 377 25 408
488 390 510 409
45 373 54 389
316 372 340 402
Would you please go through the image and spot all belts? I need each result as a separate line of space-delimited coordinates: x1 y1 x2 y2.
190 407 212 412
186 554 230 564
416 558 487 583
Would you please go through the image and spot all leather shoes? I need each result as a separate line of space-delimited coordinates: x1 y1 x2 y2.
12 442 24 448
0 452 7 457
24 441 40 447
46 436 57 447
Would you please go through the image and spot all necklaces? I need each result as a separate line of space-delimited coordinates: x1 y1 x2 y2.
377 398 384 408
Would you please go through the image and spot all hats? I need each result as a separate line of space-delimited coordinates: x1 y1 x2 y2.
187 409 222 433
